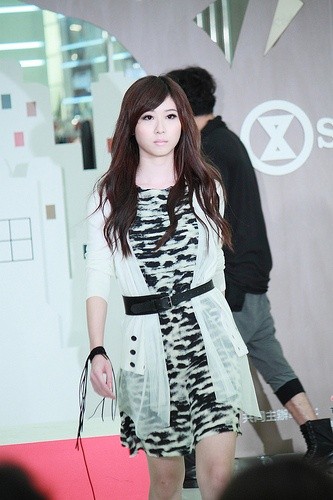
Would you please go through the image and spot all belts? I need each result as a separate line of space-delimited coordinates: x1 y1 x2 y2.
122 279 214 315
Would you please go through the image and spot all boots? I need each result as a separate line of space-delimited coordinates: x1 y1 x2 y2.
297 417 333 469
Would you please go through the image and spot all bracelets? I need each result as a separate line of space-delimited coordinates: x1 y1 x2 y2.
75 346 117 500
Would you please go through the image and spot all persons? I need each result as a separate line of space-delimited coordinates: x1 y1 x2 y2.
162 66 333 488
216 453 333 500
86 75 262 500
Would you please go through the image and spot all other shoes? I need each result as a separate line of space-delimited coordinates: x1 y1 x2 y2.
183 464 199 489
